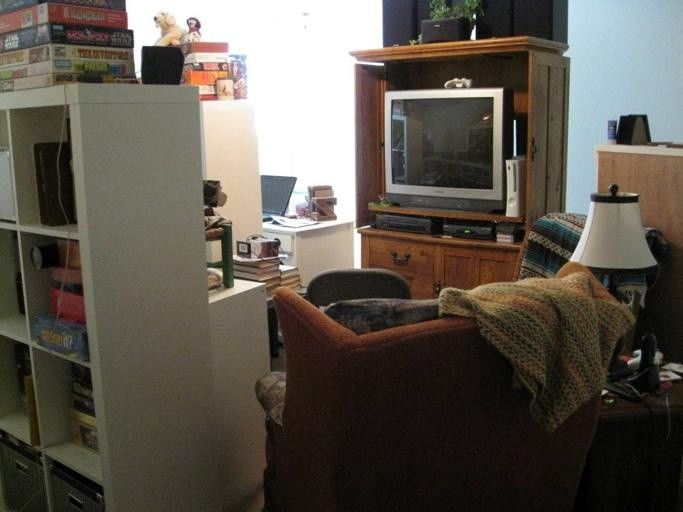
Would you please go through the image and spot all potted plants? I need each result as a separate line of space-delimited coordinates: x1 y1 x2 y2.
409 0 484 46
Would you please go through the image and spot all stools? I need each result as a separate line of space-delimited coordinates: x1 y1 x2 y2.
205 225 233 288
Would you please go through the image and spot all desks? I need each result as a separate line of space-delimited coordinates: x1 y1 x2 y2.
266 294 307 357
210 279 270 512
263 220 355 287
578 381 683 512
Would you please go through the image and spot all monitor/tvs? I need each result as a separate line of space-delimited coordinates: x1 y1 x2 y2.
383 86 514 212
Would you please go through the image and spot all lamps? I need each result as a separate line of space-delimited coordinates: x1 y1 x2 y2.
568 184 657 380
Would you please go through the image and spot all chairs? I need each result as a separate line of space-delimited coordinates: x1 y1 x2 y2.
307 268 410 307
256 262 635 512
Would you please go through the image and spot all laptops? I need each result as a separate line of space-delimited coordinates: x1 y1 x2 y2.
261 174 297 222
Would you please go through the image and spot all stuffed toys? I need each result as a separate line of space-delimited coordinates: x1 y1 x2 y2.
151 10 183 49
175 17 201 46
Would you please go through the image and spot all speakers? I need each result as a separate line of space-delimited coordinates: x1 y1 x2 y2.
382 0 569 47
140 46 184 85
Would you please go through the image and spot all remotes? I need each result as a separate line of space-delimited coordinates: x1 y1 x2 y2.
605 380 636 401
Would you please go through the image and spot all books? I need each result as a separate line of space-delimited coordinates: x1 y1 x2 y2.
227 253 304 307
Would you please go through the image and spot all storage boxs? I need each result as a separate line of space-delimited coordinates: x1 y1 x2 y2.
30 239 89 363
70 419 99 453
167 42 228 99
237 240 279 258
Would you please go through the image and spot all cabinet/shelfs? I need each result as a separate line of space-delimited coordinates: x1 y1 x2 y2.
0 84 213 512
350 38 572 301
